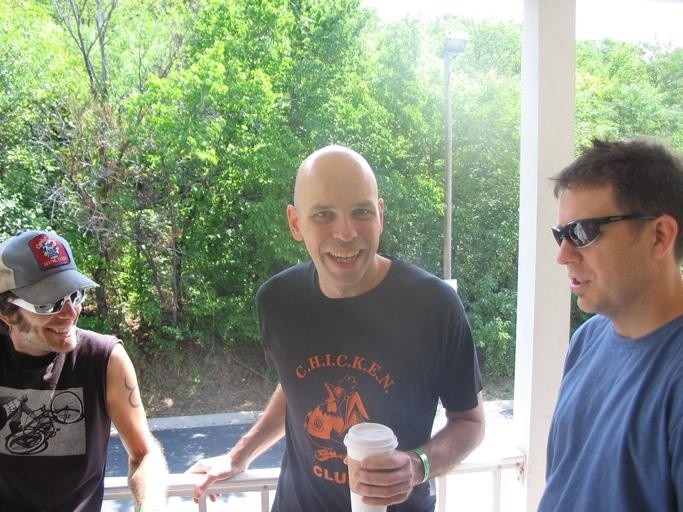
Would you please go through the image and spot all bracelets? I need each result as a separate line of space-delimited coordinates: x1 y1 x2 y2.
412 447 431 488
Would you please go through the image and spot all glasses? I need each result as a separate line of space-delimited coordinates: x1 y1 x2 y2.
552 214 662 248
7 287 85 315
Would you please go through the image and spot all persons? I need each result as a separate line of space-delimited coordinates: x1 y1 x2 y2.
536 133 683 510
181 143 488 511
0 229 171 512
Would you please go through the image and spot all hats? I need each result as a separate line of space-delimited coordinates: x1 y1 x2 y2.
0 231 99 306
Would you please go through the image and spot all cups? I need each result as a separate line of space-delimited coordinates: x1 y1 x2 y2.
343 422 399 512
